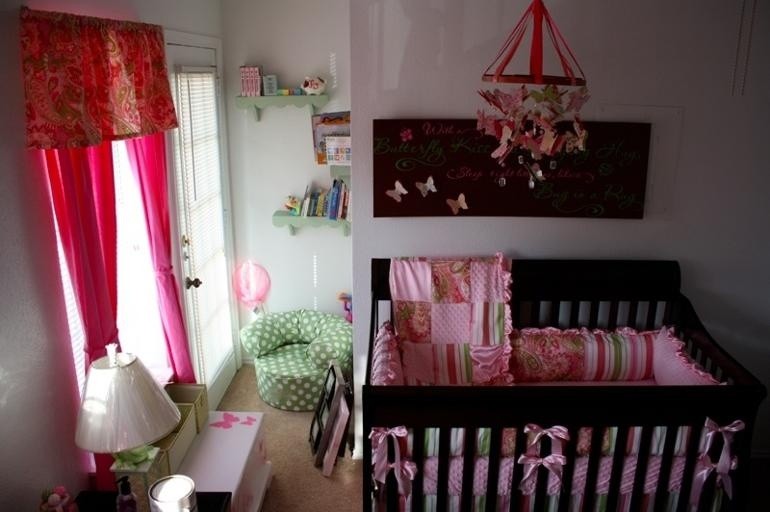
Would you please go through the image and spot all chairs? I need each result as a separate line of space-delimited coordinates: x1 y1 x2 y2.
239 309 352 412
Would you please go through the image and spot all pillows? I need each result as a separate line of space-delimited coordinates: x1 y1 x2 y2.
512 326 660 382
652 327 722 385
373 320 408 469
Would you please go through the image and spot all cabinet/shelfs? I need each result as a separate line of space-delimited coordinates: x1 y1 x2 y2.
236 94 351 238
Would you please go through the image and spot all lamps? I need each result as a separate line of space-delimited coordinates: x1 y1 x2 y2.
476 0 592 164
73 344 182 465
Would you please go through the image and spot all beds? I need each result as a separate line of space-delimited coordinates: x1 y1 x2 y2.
362 258 770 512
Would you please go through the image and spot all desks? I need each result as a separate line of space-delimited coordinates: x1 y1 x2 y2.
175 411 273 512
66 491 231 512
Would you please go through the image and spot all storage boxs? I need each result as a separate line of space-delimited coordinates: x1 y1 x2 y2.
145 403 198 474
114 450 169 511
163 383 209 432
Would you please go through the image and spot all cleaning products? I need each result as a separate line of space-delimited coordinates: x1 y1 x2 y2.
116 480 137 512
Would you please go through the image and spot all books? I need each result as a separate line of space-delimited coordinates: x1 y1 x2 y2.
240 65 261 96
300 180 350 219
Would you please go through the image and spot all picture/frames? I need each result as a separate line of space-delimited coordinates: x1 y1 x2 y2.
308 364 345 467
322 396 350 477
